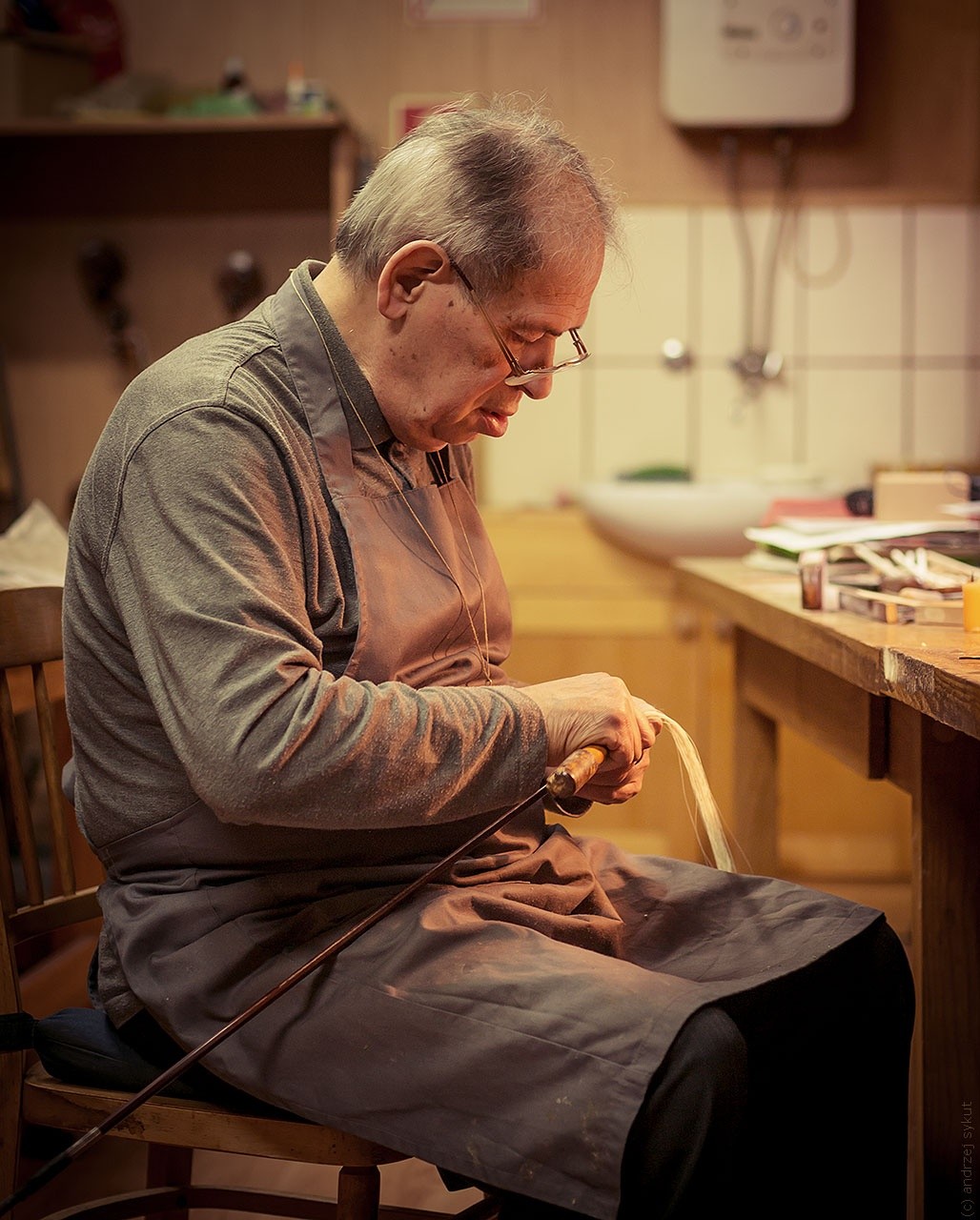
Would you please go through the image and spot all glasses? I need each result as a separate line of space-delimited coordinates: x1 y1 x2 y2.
449 253 592 387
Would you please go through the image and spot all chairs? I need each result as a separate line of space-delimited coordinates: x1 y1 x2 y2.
0 588 409 1220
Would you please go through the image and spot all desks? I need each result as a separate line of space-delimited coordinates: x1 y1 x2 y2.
671 558 980 1220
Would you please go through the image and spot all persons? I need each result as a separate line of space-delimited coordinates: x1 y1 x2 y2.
64 96 917 1220
